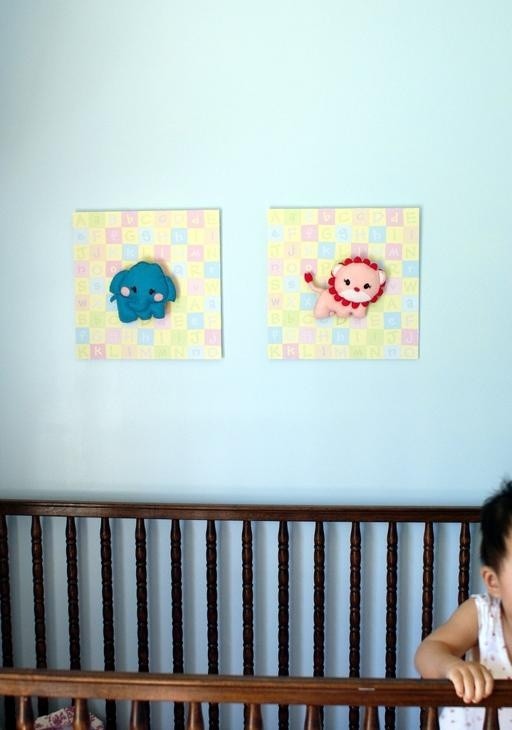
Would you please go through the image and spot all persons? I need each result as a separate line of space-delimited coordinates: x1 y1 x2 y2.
413 472 511 730
